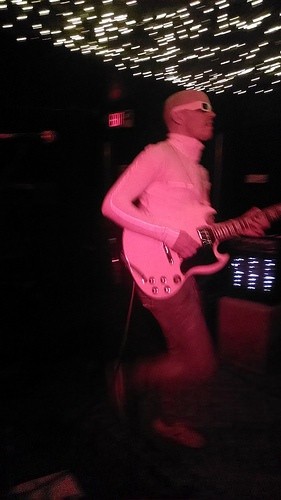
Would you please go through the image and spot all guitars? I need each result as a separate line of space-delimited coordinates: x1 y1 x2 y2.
123 203 281 300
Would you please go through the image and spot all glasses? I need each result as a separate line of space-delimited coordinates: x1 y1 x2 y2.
171 101 212 112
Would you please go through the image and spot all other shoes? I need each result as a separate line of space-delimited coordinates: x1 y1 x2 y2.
151 414 204 448
107 361 127 423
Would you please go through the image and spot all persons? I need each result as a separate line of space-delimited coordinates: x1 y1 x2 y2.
101 89 270 448
